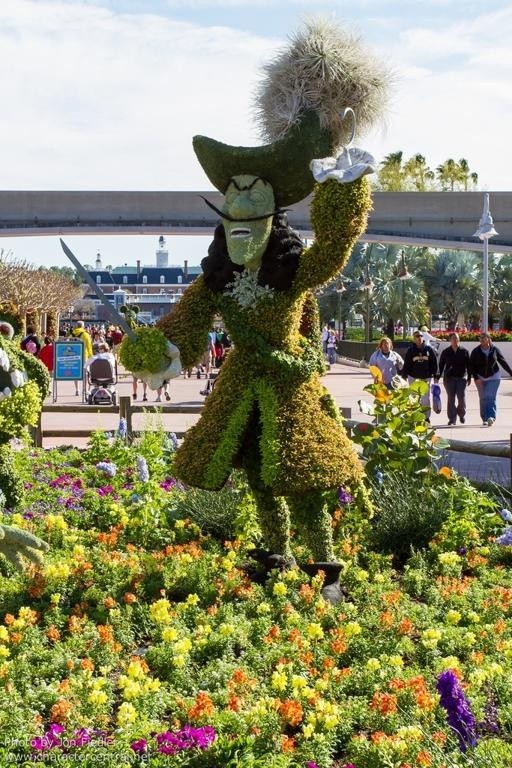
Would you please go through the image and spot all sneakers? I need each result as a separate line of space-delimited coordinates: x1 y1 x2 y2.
460 416 465 423
483 417 493 426
448 422 456 425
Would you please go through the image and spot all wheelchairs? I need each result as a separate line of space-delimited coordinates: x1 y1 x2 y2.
82 353 120 407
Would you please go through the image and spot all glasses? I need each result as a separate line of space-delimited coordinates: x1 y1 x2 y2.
415 334 423 338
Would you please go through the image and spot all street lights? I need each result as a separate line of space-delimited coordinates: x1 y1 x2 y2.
67 304 74 332
314 287 326 296
336 273 346 341
361 262 375 341
438 314 443 331
473 192 501 342
397 249 410 341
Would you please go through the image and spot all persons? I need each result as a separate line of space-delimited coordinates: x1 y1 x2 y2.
66 321 93 396
319 321 338 364
1 320 54 377
59 320 124 355
366 322 512 428
183 326 231 378
131 321 170 401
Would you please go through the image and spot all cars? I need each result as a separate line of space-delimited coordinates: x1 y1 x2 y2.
349 314 363 326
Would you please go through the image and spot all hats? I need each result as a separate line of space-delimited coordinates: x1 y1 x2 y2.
421 326 430 332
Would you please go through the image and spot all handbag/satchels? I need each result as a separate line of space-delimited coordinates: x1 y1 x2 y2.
431 384 441 414
